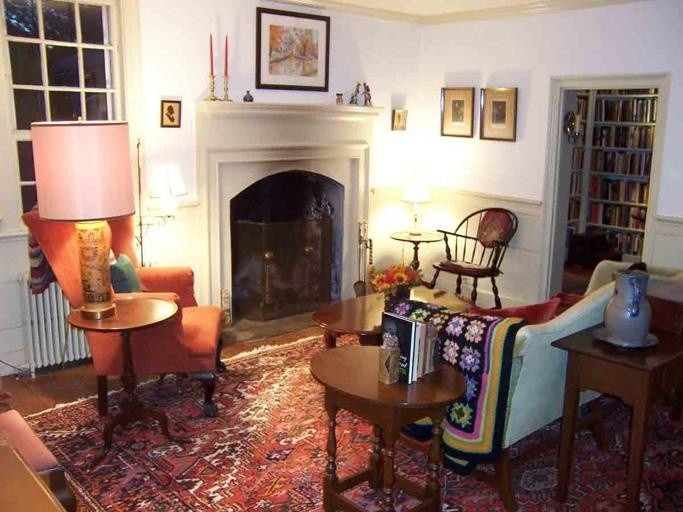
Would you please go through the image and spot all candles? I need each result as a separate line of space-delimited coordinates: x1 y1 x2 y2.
208 33 229 76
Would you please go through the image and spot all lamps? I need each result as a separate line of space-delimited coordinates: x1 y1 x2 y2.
29 116 136 320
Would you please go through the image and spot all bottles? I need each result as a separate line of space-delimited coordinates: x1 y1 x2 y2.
243 90 253 102
602 269 652 347
336 93 343 105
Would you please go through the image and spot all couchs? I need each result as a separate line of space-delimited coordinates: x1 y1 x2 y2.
389 257 681 512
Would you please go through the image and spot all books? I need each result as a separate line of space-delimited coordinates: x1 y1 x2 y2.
565 89 660 256
383 311 438 384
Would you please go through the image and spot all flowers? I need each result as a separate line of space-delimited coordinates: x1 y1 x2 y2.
370 264 422 302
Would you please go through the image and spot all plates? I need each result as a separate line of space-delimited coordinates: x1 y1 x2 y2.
593 326 660 350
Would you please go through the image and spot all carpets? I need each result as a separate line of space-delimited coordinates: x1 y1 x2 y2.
24 334 682 511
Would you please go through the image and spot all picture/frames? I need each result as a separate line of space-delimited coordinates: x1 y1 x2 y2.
439 86 517 142
253 7 330 93
159 100 182 128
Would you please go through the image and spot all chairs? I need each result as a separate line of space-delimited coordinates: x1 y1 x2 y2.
21 202 228 420
429 207 519 308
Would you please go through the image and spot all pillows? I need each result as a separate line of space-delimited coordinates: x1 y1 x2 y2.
108 252 143 294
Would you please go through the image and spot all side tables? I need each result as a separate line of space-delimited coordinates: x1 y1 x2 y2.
390 232 443 270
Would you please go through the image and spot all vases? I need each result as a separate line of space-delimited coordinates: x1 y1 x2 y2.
383 292 410 311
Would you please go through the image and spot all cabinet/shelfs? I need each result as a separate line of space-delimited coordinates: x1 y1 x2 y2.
564 88 656 236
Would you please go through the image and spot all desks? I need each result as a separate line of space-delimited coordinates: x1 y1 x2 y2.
67 298 196 475
308 343 466 510
549 321 683 511
